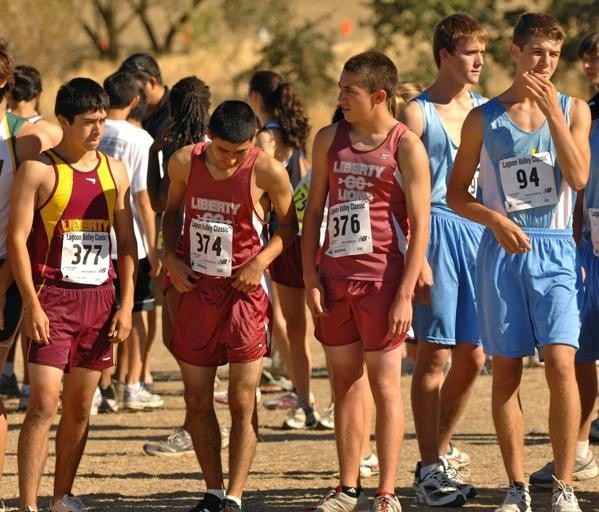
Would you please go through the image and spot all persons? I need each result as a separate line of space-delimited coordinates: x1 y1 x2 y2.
0 6 599 512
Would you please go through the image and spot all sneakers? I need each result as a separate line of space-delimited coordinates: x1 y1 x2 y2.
529 450 599 485
370 492 403 512
218 498 244 512
188 491 223 512
551 475 582 512
412 441 476 507
358 451 379 477
263 392 335 429
49 492 87 512
494 482 532 512
214 387 261 405
0 374 165 416
144 425 195 456
304 486 371 512
